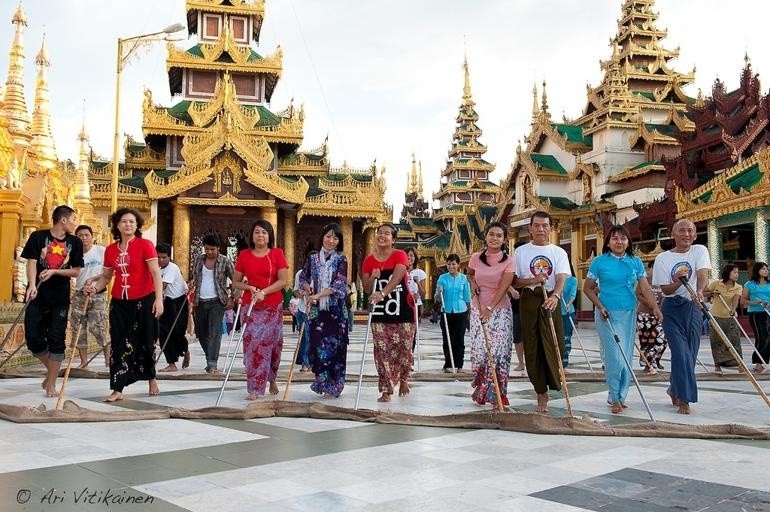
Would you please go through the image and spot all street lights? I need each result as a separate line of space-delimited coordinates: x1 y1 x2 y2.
107 23 185 300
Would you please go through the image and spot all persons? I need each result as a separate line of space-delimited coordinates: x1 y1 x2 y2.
581 225 663 414
515 210 570 414
155 240 192 372
20 204 86 396
231 219 290 402
650 220 711 416
364 224 417 403
185 235 237 371
300 223 350 401
86 209 167 401
467 223 515 411
72 222 108 369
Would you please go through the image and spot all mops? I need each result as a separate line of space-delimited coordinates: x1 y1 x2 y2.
0 207 769 442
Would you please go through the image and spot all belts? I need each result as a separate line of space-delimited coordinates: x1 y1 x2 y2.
198 297 220 303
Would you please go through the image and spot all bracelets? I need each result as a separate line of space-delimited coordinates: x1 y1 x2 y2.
93 287 98 294
261 290 265 296
